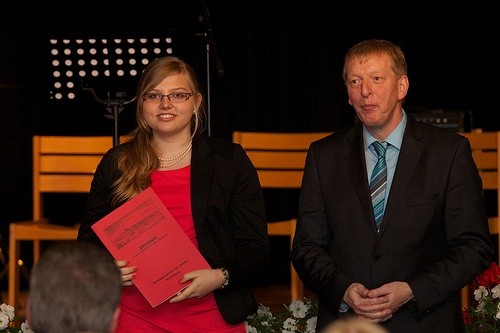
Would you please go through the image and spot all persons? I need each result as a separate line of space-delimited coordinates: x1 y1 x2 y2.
290 39 494 333
323 318 388 333
27 243 123 332
77 56 273 333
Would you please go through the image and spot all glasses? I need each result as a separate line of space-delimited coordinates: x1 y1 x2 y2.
143 93 193 103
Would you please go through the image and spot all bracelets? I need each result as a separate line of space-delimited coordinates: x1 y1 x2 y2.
217 267 230 289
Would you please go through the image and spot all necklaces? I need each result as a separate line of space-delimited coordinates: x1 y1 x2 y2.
157 140 192 167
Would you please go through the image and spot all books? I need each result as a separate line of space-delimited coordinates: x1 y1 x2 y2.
91 187 212 308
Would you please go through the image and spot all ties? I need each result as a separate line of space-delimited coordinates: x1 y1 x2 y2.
370 141 388 234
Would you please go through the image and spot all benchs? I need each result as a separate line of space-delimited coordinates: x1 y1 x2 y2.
9 132 500 326
